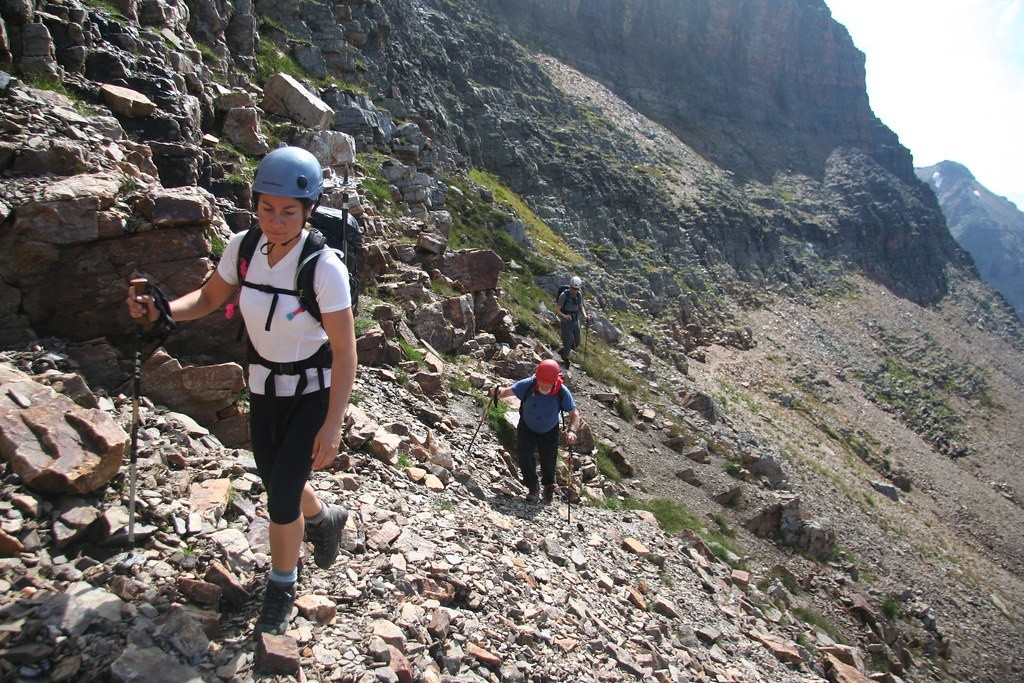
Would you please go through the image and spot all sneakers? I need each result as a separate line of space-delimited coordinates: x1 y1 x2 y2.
542 484 554 505
526 483 540 502
253 580 296 641
303 503 349 571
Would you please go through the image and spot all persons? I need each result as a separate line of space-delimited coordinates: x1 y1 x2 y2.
128 147 357 639
556 277 590 368
490 359 580 502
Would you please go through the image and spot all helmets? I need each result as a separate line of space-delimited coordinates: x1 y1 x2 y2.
252 146 324 202
570 276 582 288
535 359 560 385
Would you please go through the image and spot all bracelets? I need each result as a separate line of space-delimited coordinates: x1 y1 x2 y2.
498 388 501 394
568 429 577 434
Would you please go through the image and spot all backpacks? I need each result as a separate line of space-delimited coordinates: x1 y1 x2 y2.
234 205 363 331
556 285 581 308
